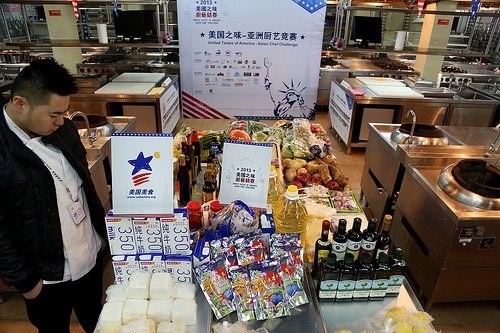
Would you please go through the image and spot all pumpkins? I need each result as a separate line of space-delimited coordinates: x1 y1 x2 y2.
228 130 268 142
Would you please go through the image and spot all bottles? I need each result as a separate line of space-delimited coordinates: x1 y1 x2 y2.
268 165 287 206
385 246 408 297
173 126 222 231
334 252 359 303
273 184 310 254
369 252 392 301
312 220 332 280
317 253 340 303
346 217 364 261
373 214 392 261
332 219 348 262
352 252 376 302
359 217 379 258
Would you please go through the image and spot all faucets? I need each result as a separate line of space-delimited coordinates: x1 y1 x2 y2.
457 80 467 92
482 126 500 158
493 82 499 94
68 110 99 148
404 109 418 137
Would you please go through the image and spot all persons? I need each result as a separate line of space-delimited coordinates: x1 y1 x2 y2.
0 55 110 333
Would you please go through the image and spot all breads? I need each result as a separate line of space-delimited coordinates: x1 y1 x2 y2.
95 270 198 333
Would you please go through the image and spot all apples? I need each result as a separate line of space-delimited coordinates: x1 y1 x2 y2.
290 173 340 191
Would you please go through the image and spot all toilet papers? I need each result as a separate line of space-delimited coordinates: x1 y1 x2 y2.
393 30 406 52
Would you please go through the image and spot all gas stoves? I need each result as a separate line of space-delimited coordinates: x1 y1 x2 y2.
319 55 349 69
370 58 414 71
440 64 469 74
148 53 180 65
24 56 55 63
82 50 131 65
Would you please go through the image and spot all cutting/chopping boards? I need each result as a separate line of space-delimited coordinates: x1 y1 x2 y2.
94 82 157 95
112 72 166 83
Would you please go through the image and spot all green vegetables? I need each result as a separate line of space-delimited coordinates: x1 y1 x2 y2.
249 121 306 158
199 130 226 149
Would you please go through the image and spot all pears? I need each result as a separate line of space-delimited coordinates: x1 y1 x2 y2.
283 157 320 181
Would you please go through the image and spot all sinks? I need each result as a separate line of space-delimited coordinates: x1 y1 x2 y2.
70 113 109 129
491 93 500 98
398 122 444 138
455 92 493 101
451 158 500 198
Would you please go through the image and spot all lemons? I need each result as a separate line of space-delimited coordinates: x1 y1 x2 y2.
385 308 432 333
